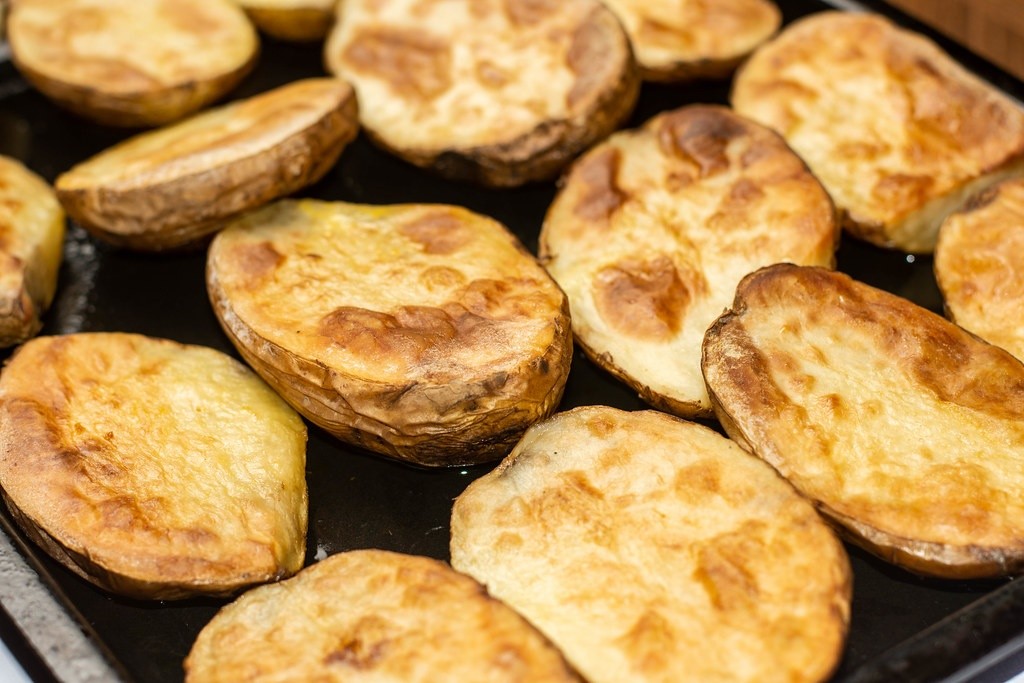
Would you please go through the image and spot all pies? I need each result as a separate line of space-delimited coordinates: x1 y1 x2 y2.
0 0 1024 683
